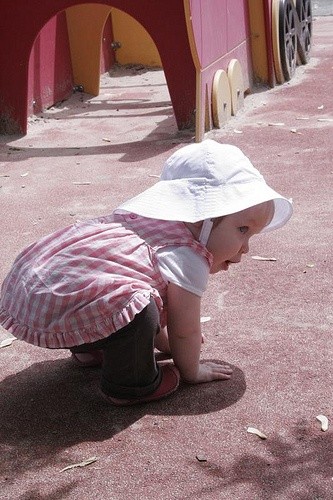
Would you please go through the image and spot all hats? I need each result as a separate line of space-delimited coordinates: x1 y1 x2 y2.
117 139 293 234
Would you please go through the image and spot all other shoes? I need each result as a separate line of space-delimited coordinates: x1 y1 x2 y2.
72 348 105 367
98 364 179 406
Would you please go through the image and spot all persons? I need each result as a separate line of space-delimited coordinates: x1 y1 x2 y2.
0 139 293 405
